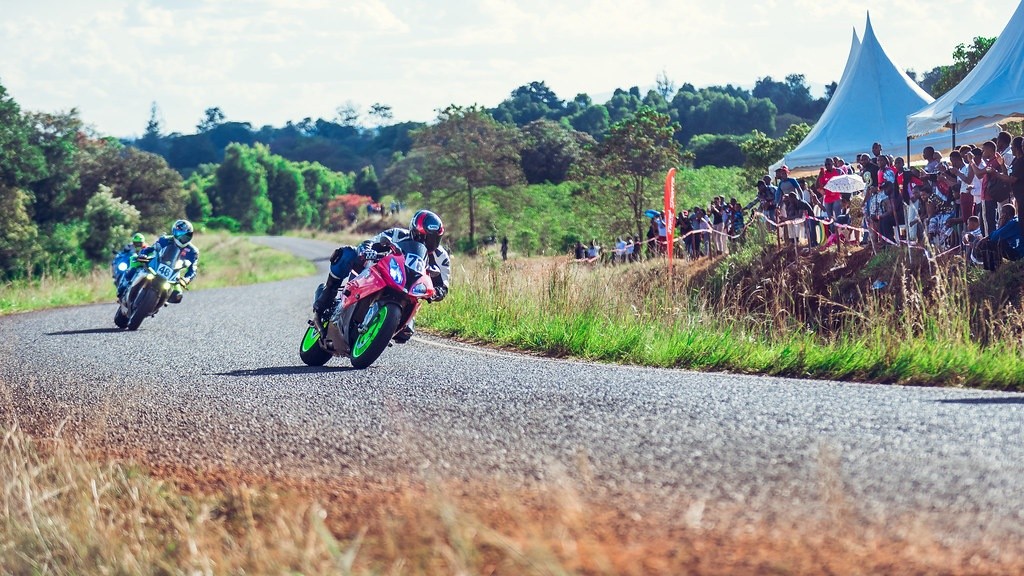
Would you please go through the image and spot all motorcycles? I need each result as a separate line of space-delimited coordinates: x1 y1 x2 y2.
297 235 443 370
111 247 192 331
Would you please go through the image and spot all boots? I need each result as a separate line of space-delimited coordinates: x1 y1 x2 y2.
312 271 343 312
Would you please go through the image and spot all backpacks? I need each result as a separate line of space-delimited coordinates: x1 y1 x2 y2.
984 163 1010 203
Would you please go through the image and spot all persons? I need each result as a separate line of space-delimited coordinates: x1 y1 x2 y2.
500 234 509 260
367 200 406 218
615 234 640 264
675 196 746 260
575 241 601 265
645 211 667 260
857 121 1024 274
112 220 200 303
313 210 451 344
351 212 357 225
740 156 856 250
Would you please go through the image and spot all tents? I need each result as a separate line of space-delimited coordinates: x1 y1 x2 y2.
768 11 1003 185
907 0 1024 166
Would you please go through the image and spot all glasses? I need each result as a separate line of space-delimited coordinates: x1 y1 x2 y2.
825 163 831 166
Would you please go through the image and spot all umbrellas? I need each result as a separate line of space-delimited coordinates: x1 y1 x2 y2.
823 175 867 193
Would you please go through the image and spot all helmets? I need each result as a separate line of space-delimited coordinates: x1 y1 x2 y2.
409 209 445 251
129 233 145 243
172 220 194 248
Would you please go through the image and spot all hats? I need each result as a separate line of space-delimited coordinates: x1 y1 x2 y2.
774 165 790 173
933 151 942 159
763 176 771 181
754 180 764 187
